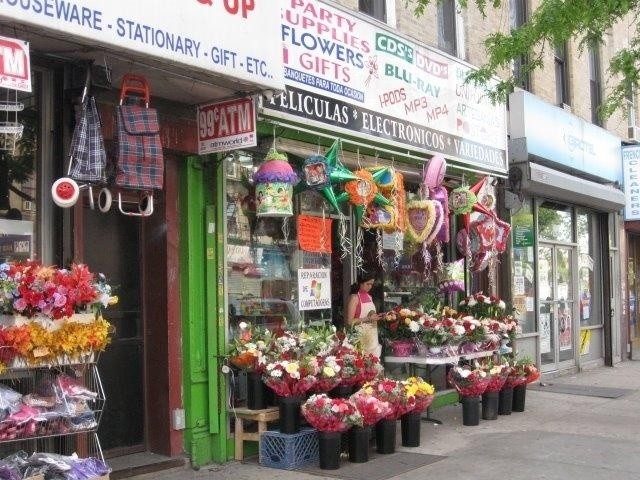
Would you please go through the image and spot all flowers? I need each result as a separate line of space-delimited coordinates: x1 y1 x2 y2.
447 350 540 398
301 376 435 431
383 288 525 349
229 313 382 395
0 256 120 388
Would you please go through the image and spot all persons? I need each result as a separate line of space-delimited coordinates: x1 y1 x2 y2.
348 275 385 358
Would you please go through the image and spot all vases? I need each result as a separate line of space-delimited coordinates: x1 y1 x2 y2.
498 387 512 415
402 412 419 448
513 384 526 412
482 391 497 420
390 342 414 357
377 418 396 454
279 396 298 434
462 397 479 427
349 424 368 462
247 371 267 409
318 430 340 467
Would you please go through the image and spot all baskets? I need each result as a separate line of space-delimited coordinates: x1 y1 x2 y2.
259 428 319 471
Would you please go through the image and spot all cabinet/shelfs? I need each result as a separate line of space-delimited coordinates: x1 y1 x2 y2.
0 362 112 478
